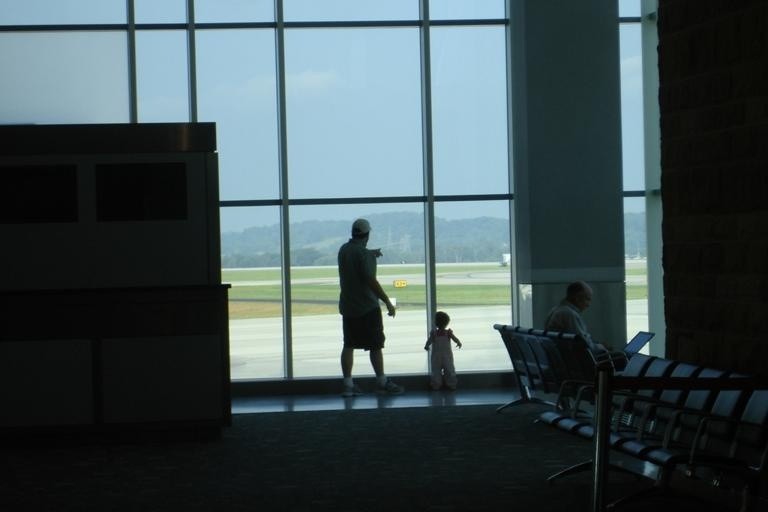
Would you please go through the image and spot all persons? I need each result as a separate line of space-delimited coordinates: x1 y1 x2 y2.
336 218 406 395
424 312 463 391
541 280 613 404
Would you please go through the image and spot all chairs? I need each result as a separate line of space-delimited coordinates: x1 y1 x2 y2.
492 323 768 512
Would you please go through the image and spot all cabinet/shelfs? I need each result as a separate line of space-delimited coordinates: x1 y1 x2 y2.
0 124 233 442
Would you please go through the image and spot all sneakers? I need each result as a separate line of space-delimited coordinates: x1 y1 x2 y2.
375 383 404 394
344 384 363 396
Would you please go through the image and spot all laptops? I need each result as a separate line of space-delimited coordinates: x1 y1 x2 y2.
623 330 656 358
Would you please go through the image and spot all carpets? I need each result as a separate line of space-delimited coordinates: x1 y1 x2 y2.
0 398 768 512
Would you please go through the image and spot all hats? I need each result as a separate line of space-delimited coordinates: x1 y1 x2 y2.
352 219 372 235
433 385 456 390
